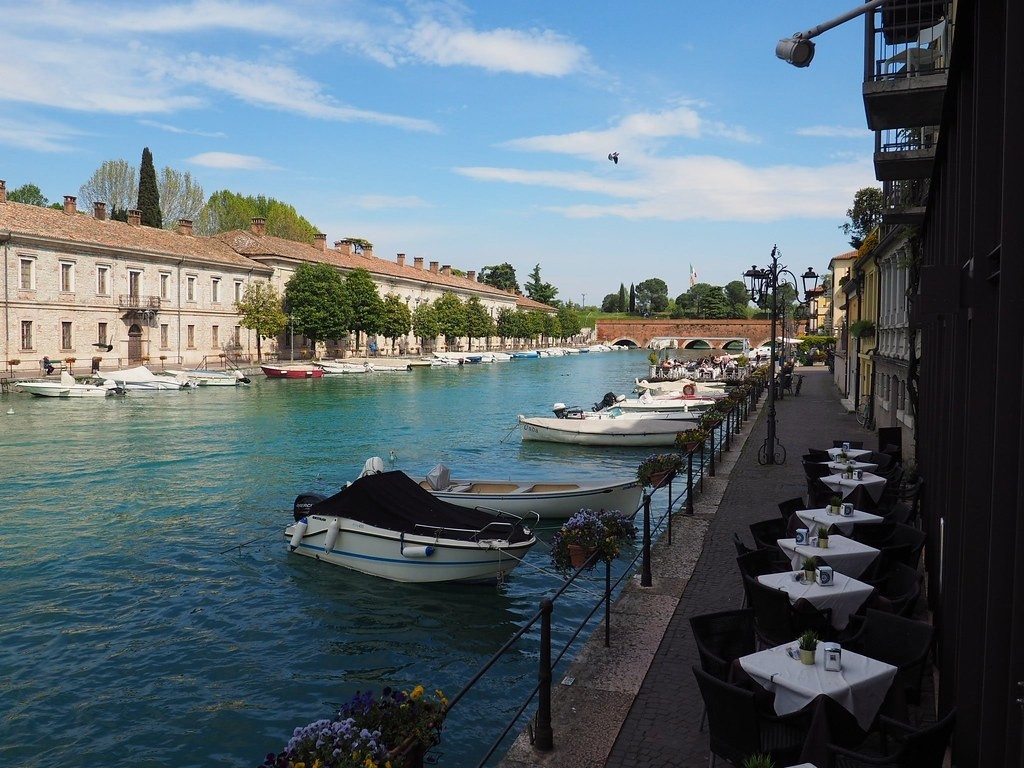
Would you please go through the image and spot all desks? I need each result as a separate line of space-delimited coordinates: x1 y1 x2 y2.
793 508 884 536
757 569 875 631
825 448 874 462
819 460 879 474
819 471 887 510
777 533 881 579
739 634 899 733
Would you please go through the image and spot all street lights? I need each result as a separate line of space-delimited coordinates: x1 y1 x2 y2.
776 293 805 399
137 310 156 364
743 244 820 467
284 315 301 363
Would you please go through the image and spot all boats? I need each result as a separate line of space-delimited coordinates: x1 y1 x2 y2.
719 347 776 361
260 365 324 378
517 415 700 447
313 345 628 374
633 378 729 401
341 457 644 525
552 403 724 429
164 369 251 387
92 366 200 391
285 469 540 583
15 371 122 397
591 392 716 414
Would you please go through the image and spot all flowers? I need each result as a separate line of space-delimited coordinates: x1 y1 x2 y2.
730 365 770 399
674 428 708 449
261 682 448 768
702 411 724 428
548 507 638 575
714 399 733 409
635 452 686 491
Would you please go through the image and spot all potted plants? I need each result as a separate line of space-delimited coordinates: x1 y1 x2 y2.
831 496 842 513
852 319 875 338
799 630 817 665
846 466 853 478
818 528 828 547
803 555 816 582
841 452 846 463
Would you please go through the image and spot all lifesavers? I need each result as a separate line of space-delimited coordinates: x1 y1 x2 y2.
363 362 368 367
682 385 695 395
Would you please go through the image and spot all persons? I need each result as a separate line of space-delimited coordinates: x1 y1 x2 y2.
43 356 54 375
370 339 376 356
661 352 770 382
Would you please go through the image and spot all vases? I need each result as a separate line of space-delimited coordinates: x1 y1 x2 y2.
720 407 730 413
382 724 442 767
649 466 676 488
685 440 702 453
567 544 600 569
707 418 716 428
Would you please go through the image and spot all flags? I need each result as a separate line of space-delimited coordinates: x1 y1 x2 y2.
689 263 697 289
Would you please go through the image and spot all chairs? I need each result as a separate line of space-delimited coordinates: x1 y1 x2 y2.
687 441 955 768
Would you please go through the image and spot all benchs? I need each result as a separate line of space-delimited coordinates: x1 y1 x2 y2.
42 358 67 375
445 486 472 492
511 484 534 494
